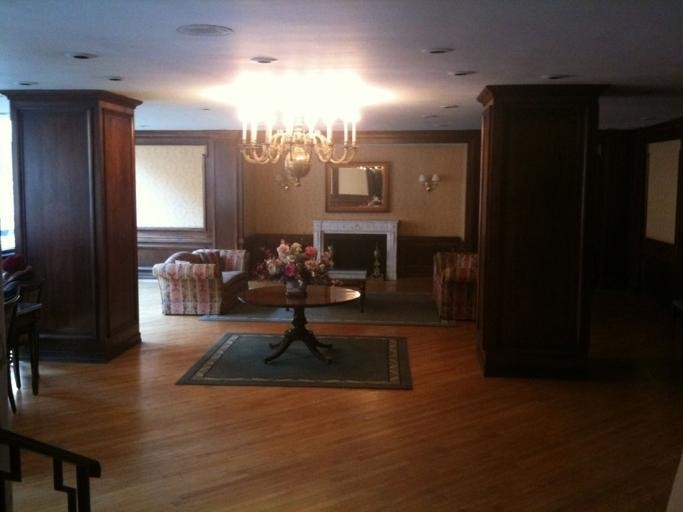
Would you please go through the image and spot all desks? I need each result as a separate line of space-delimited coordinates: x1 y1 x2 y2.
233 284 361 365
326 269 370 313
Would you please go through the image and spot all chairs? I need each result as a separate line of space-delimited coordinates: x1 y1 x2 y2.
0 249 47 415
431 250 480 325
148 248 252 317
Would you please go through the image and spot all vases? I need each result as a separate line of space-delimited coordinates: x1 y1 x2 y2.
282 276 311 299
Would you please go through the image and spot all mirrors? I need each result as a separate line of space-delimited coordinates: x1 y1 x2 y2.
324 160 390 214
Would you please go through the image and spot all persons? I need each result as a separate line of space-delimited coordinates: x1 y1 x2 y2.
2 252 34 298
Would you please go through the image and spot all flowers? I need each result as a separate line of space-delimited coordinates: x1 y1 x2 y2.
254 237 335 284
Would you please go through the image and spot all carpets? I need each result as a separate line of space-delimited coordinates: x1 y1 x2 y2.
196 290 481 329
174 331 413 392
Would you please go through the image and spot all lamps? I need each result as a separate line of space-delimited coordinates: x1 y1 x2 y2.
417 173 440 192
236 117 359 191
